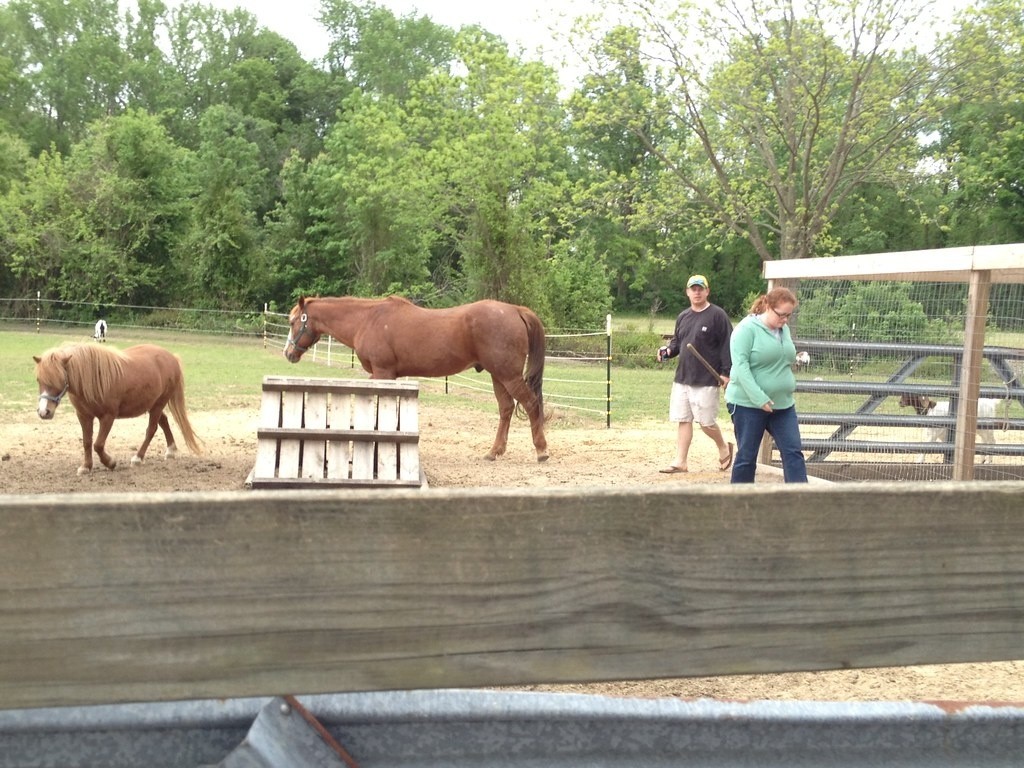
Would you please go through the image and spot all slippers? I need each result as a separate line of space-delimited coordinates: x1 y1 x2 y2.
658 465 688 474
718 442 733 472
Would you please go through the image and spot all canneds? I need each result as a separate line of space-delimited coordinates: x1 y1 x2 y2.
660 346 668 361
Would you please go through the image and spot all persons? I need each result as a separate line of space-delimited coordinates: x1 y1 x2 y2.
656 274 733 474
724 286 808 484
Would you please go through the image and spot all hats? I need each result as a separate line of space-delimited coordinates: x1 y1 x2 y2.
686 275 707 290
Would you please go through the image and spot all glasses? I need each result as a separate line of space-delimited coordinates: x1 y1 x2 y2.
772 309 792 318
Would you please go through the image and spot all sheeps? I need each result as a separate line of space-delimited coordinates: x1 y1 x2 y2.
91 319 108 343
899 390 1003 467
794 350 811 373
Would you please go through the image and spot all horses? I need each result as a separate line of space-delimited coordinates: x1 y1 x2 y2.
284 293 552 464
31 343 208 474
